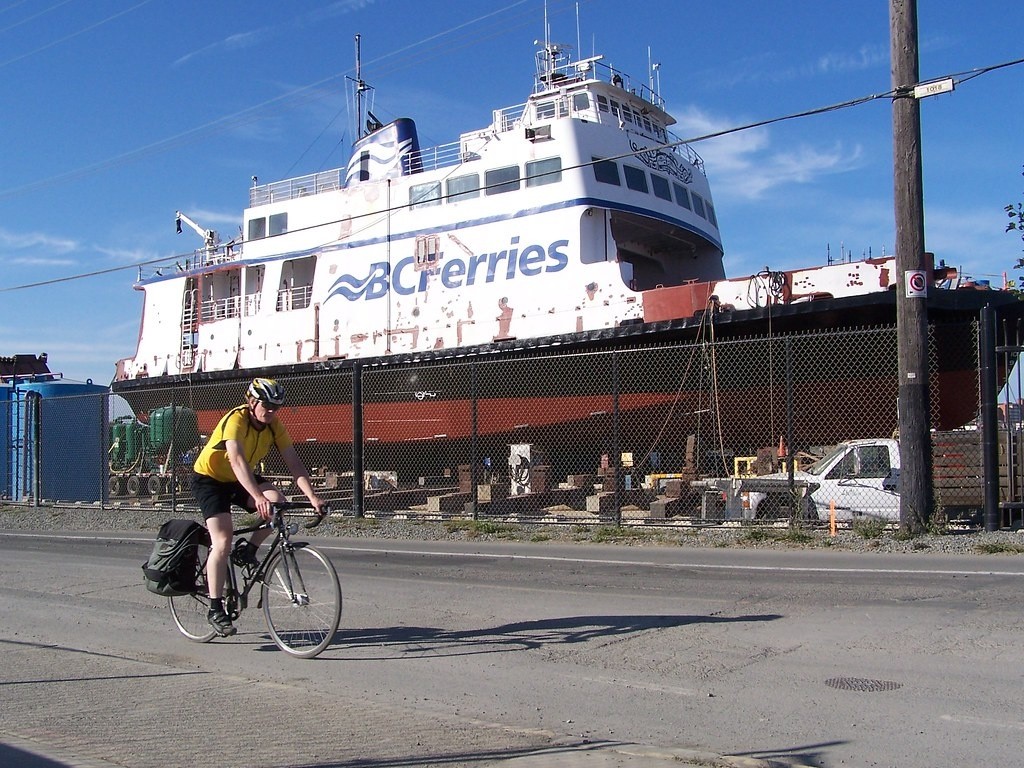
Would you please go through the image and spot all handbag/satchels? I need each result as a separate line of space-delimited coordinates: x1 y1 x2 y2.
142 518 206 596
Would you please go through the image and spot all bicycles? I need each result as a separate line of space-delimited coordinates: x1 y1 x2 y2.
167 501 343 660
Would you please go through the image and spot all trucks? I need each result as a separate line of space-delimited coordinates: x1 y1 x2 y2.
740 426 1024 525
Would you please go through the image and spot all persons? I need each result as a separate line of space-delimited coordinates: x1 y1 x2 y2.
192 377 329 637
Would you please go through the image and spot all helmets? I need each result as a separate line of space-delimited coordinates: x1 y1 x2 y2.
244 377 286 406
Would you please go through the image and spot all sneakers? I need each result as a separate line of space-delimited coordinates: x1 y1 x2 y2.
229 545 263 580
208 609 237 637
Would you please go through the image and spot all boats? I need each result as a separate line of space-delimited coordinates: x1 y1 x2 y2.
104 0 1024 471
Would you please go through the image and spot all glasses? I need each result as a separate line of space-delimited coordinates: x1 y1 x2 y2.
258 399 281 411
